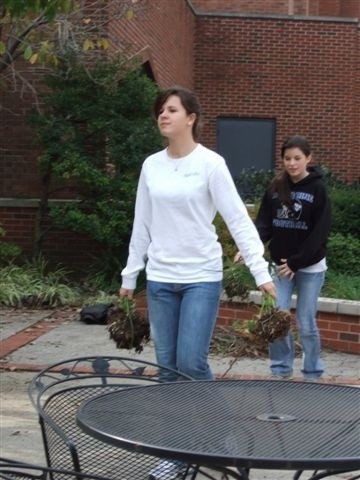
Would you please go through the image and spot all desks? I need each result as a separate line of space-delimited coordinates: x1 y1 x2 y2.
76 377 360 480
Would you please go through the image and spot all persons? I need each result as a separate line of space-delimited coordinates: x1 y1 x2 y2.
234 134 331 382
118 87 279 480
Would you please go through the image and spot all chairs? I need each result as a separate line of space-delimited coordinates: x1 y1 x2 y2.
291 412 360 480
26 354 255 480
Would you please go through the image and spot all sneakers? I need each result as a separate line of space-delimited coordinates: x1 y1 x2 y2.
147 458 196 479
